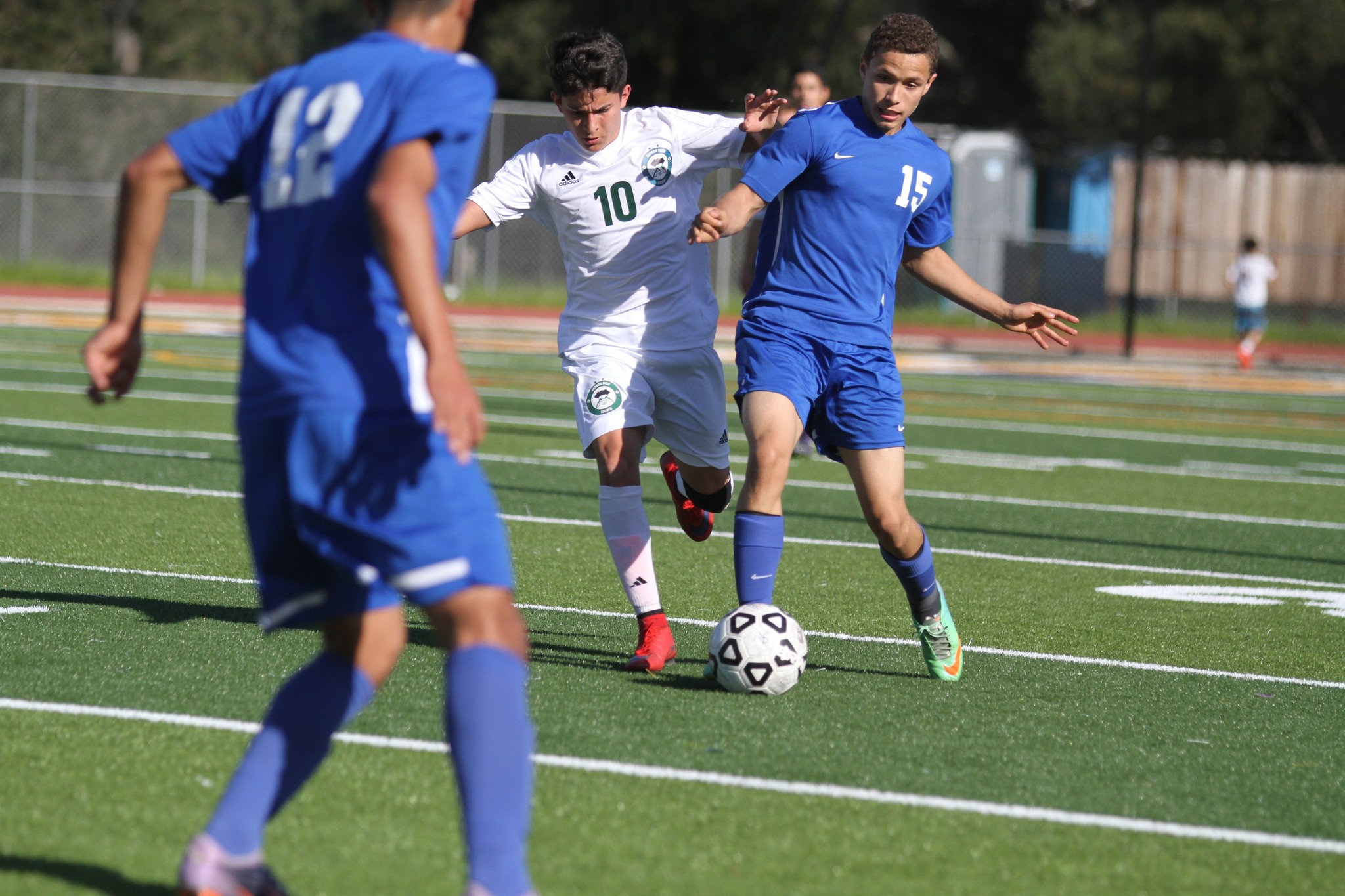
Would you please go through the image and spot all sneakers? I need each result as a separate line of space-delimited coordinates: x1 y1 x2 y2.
181 831 288 896
911 580 963 681
660 450 714 542
621 623 679 674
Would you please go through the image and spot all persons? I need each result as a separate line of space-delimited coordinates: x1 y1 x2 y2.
1222 233 1286 371
444 30 790 670
79 0 535 896
734 70 834 301
692 12 1083 682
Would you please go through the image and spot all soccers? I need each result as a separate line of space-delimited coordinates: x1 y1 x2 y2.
708 602 808 696
